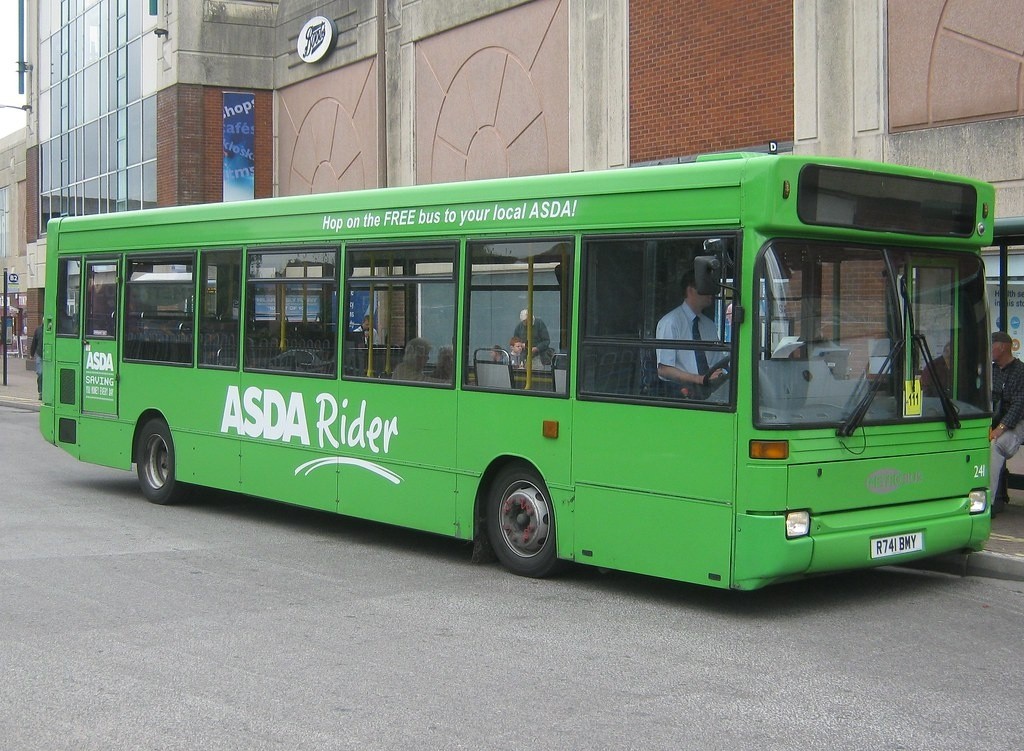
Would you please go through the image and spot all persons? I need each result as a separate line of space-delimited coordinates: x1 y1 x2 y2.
392 338 452 384
990 332 1024 518
655 271 729 400
354 316 378 343
725 304 732 323
30 313 44 400
431 345 453 379
509 337 525 368
514 310 551 370
489 345 507 361
921 342 952 398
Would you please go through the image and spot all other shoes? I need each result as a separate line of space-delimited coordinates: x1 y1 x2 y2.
38 391 42 400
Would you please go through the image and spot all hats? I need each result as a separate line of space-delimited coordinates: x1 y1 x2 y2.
992 332 1012 343
520 310 528 321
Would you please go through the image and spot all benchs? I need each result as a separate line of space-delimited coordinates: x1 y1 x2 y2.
110 309 367 373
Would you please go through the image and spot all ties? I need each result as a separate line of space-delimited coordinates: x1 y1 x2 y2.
692 315 710 375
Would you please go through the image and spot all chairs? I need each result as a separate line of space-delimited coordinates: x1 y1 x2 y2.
639 300 672 393
473 347 516 389
550 353 567 392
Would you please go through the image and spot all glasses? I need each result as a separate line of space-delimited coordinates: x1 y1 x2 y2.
726 313 732 315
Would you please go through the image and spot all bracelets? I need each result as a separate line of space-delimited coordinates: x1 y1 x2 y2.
999 423 1007 430
